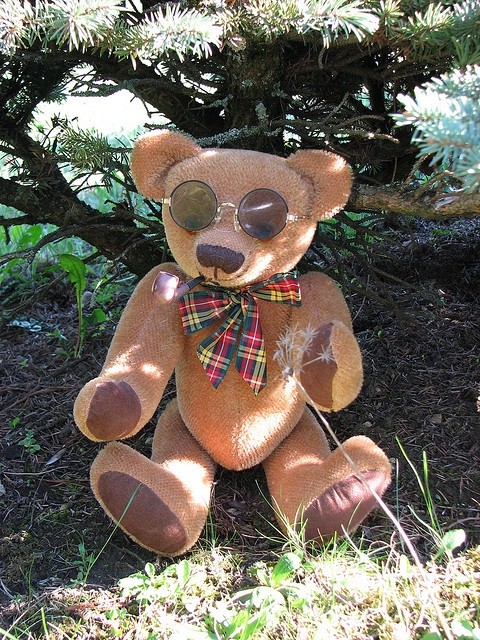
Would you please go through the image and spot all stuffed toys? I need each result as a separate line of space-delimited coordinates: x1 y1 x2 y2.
72 127 392 557
